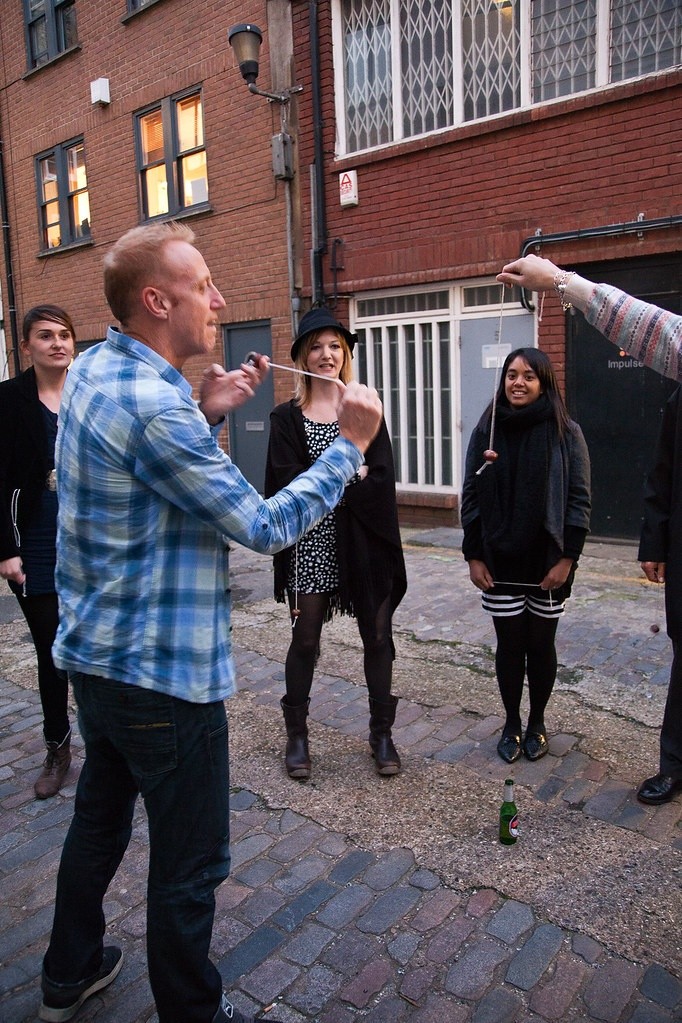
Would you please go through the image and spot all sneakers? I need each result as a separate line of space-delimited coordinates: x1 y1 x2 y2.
37 946 124 1022
211 994 282 1023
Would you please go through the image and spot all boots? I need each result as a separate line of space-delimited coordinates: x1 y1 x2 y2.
280 694 311 777
34 726 72 799
368 694 401 775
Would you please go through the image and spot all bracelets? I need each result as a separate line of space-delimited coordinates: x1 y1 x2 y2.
553 268 576 312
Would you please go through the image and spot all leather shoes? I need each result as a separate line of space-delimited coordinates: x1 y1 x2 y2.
497 726 522 763
637 772 682 804
524 725 548 761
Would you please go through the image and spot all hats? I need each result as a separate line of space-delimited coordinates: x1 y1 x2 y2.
291 308 359 361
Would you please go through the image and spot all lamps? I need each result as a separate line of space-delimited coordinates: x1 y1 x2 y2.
227 24 291 103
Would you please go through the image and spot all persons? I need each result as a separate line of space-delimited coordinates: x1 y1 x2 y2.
460 347 593 763
38 217 384 1023
0 304 77 802
264 307 403 778
496 252 682 806
